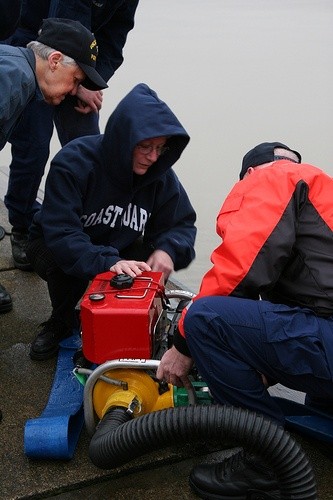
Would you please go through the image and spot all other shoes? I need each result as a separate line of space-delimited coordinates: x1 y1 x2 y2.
189 445 281 499
10 232 31 266
31 309 81 360
0 282 13 313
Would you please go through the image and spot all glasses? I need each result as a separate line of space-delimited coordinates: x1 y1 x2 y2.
136 140 167 159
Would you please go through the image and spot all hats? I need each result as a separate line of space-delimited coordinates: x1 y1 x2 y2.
38 17 109 91
240 142 301 180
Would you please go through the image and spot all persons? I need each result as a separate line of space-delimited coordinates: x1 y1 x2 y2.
1 0 139 270
0 15 110 311
29 83 198 360
155 141 333 500
